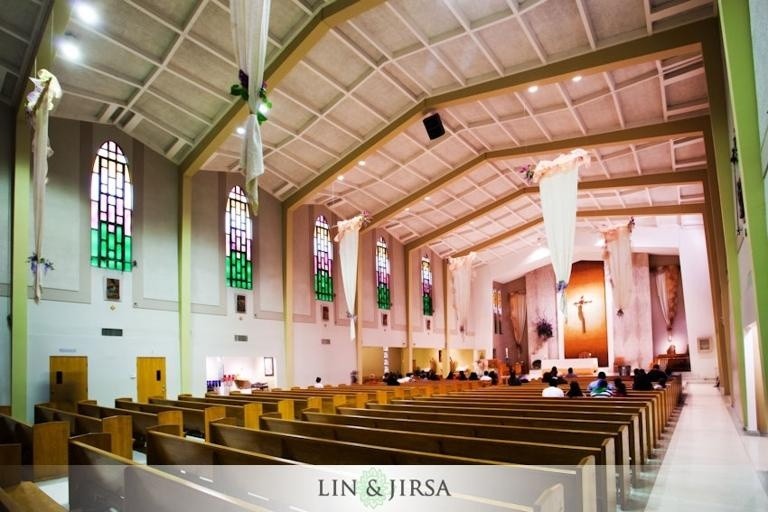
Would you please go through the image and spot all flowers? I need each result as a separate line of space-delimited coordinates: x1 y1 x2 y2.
521 164 534 183
26 251 55 295
230 70 272 125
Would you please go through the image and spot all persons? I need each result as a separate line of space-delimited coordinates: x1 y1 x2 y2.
587 371 612 390
565 381 586 397
589 380 612 398
350 370 358 384
508 369 522 386
592 369 597 376
613 377 627 396
565 367 577 378
314 377 323 388
633 363 672 389
541 366 567 384
542 379 564 397
381 367 499 386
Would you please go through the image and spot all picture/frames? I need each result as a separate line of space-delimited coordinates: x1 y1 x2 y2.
104 277 121 301
426 318 431 329
234 293 246 313
381 313 388 325
321 305 329 322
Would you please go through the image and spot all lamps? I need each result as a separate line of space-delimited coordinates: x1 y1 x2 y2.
422 112 446 141
